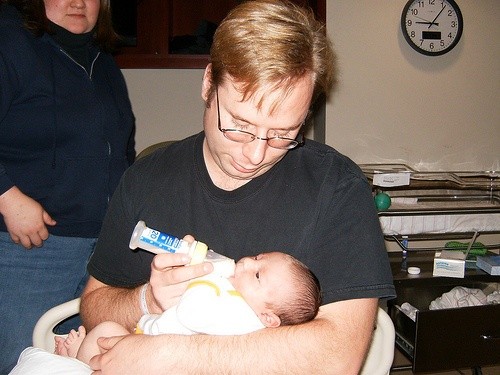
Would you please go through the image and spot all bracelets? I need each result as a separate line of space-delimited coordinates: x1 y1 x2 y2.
140 282 150 315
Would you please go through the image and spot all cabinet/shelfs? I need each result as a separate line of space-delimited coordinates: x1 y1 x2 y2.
358 163 500 375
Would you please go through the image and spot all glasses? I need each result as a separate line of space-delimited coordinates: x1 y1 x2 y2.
216 86 304 150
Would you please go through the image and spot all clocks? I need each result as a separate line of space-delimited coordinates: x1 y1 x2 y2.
400 0 464 57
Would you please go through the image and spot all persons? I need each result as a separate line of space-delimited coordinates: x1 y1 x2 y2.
0 0 138 375
80 0 397 375
55 251 323 364
